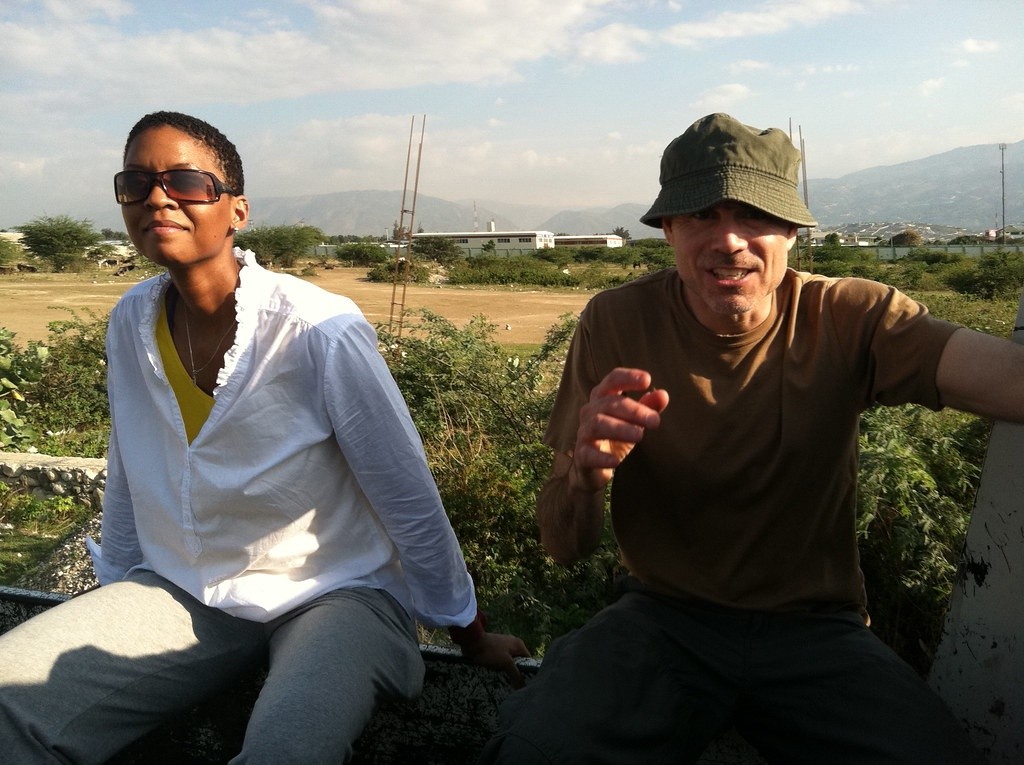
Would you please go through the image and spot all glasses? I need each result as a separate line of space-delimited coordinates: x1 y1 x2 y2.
113 168 232 205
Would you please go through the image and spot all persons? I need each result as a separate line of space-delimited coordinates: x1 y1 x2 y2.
1 111 530 765
488 113 1024 765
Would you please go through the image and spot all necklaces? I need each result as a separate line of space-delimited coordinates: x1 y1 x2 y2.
185 307 236 386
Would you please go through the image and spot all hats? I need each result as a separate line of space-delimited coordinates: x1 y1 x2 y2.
639 112 818 228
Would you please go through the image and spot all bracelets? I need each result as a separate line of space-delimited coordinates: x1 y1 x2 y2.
449 607 488 644
